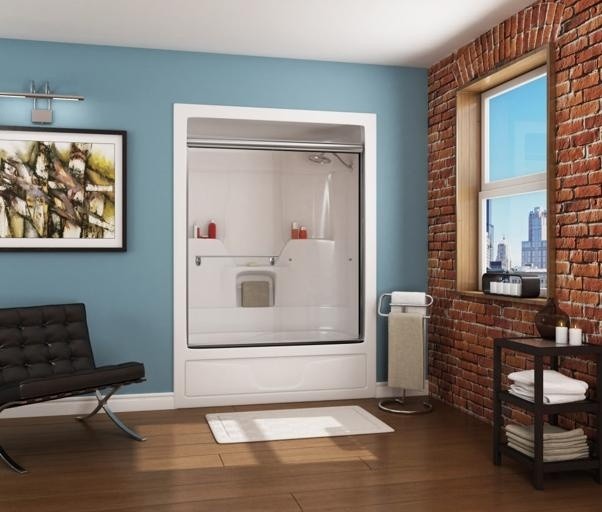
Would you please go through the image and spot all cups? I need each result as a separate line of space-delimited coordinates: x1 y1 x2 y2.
489 281 521 296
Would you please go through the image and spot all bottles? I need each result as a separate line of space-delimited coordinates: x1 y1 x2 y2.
291 221 307 239
194 222 201 238
208 218 217 239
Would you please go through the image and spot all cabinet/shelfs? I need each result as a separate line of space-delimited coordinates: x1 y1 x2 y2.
491 333 602 491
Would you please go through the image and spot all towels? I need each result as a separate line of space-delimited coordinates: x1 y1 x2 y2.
386 288 429 393
498 368 591 463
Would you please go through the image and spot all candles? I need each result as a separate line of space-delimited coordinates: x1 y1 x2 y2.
554 325 583 345
488 279 521 296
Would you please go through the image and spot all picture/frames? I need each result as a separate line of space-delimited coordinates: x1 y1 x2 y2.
0 124 126 252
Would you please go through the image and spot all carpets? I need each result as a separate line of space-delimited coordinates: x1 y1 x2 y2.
204 405 396 445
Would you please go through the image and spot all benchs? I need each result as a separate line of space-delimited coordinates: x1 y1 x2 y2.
0 302 148 473
